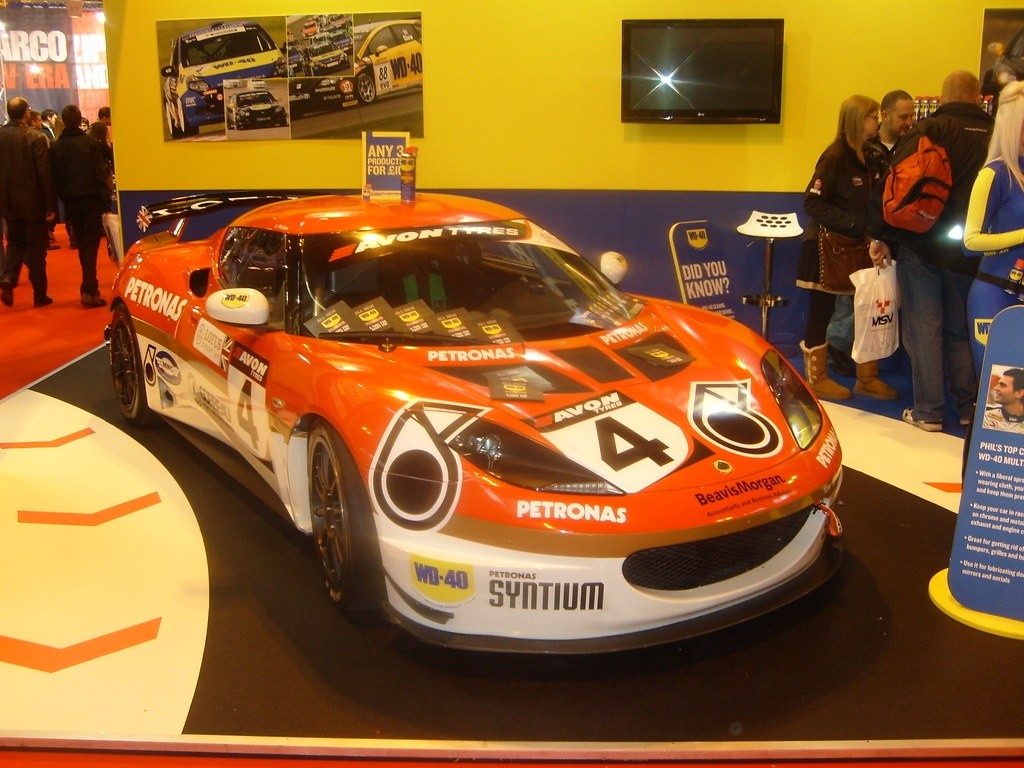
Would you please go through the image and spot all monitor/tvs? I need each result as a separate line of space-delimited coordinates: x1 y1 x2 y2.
620 19 784 123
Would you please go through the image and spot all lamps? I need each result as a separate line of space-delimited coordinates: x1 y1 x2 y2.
68 0 83 19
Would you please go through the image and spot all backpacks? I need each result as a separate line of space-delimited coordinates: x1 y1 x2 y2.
880 124 953 233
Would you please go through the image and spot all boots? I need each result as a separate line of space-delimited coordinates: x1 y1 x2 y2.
799 340 851 401
854 360 899 400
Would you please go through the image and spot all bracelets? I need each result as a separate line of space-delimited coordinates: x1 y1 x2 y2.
870 238 883 242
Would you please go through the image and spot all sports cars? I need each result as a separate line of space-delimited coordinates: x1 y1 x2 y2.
101 187 845 656
353 19 423 106
226 87 287 131
159 19 289 139
283 13 353 77
288 77 357 120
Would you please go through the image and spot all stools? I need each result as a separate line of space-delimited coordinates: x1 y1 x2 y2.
737 211 805 359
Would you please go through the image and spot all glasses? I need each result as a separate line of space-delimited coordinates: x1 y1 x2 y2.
28 107 32 112
866 114 880 120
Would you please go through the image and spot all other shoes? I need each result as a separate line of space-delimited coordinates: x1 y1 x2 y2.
70 240 78 249
826 344 856 376
48 230 56 242
902 408 943 432
34 296 52 306
959 418 971 425
81 293 106 306
2 275 14 305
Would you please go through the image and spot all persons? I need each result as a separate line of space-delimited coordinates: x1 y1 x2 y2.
982 368 1024 434
0 96 117 307
823 90 915 377
963 79 1024 433
796 96 901 400
867 69 993 433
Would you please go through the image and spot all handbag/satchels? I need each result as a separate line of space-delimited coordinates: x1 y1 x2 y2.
849 259 899 364
817 229 874 294
